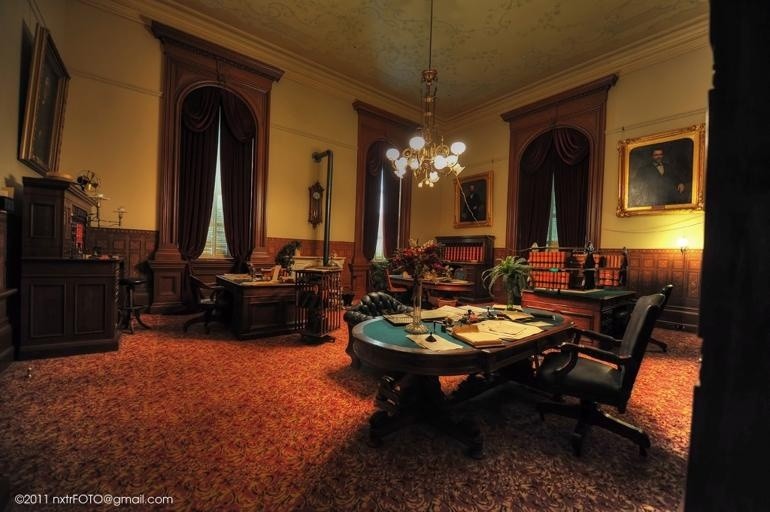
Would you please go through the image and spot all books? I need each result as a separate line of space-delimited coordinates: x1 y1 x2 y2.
444 246 482 262
526 252 624 288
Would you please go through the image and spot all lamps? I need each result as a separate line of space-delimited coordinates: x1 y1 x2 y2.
675 235 688 255
385 0 467 190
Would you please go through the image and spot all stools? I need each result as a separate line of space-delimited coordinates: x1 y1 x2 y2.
118 277 151 335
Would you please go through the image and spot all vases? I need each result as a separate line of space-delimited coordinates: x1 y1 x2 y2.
405 269 429 334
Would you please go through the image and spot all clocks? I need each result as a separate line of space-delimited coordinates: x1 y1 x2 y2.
307 179 325 230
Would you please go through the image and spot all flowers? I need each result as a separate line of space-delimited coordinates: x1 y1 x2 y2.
384 236 452 282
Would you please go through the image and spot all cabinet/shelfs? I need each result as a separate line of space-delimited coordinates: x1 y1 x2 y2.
434 235 495 304
16 171 127 360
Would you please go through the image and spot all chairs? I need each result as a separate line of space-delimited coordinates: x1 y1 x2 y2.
368 259 408 300
185 274 227 335
618 282 675 354
524 280 675 457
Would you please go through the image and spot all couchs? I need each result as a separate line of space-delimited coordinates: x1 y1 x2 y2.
342 289 415 355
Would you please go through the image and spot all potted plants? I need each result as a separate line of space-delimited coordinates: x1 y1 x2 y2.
480 252 534 313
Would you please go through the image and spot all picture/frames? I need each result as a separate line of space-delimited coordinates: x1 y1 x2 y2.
15 20 72 177
452 170 496 230
615 121 706 217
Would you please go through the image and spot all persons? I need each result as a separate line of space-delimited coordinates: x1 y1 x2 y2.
35 77 54 139
635 147 690 206
462 185 481 219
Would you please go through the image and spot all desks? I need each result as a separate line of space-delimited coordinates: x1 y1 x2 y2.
520 285 640 335
215 272 310 341
389 273 476 310
352 302 577 460
